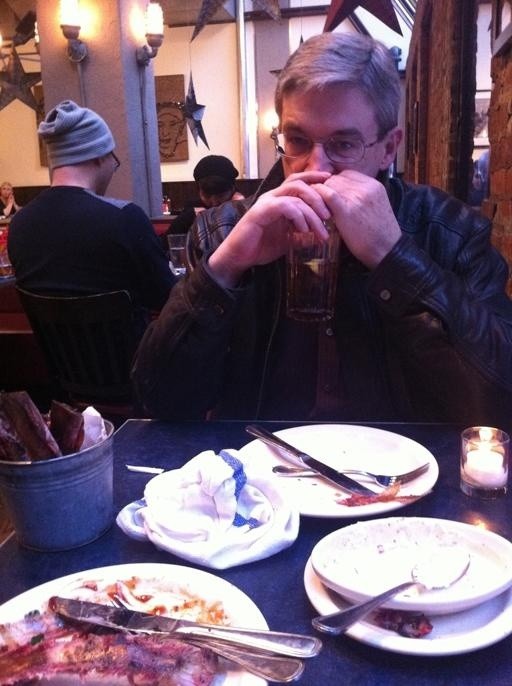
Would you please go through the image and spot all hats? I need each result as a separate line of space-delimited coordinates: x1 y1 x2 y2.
38 100 115 169
193 155 239 184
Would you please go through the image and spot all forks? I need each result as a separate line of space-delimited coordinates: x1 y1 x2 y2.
102 596 305 685
269 459 432 484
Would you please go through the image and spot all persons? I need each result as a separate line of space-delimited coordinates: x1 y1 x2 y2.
0 181 22 220
156 102 189 160
130 32 511 420
8 103 177 401
162 154 249 245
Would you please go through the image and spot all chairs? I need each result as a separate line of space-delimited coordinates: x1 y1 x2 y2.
13 281 135 408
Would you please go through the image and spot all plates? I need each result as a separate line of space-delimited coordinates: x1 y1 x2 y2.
302 558 511 658
236 424 439 520
2 564 270 684
312 515 511 614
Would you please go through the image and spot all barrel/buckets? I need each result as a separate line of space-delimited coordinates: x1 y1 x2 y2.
0 419 114 552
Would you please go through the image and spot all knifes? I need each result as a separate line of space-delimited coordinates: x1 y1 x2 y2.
42 594 320 663
245 421 379 499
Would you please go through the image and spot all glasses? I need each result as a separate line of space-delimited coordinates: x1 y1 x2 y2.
273 132 378 163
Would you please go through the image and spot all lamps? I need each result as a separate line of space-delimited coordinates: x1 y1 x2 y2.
59 0 88 63
135 3 164 66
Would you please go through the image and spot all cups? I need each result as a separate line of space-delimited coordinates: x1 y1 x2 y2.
163 199 171 215
286 210 341 327
167 232 190 270
459 427 508 501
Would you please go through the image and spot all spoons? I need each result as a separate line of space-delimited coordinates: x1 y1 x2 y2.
311 544 471 637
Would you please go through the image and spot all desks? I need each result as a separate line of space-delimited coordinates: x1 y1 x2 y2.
2 415 510 685
